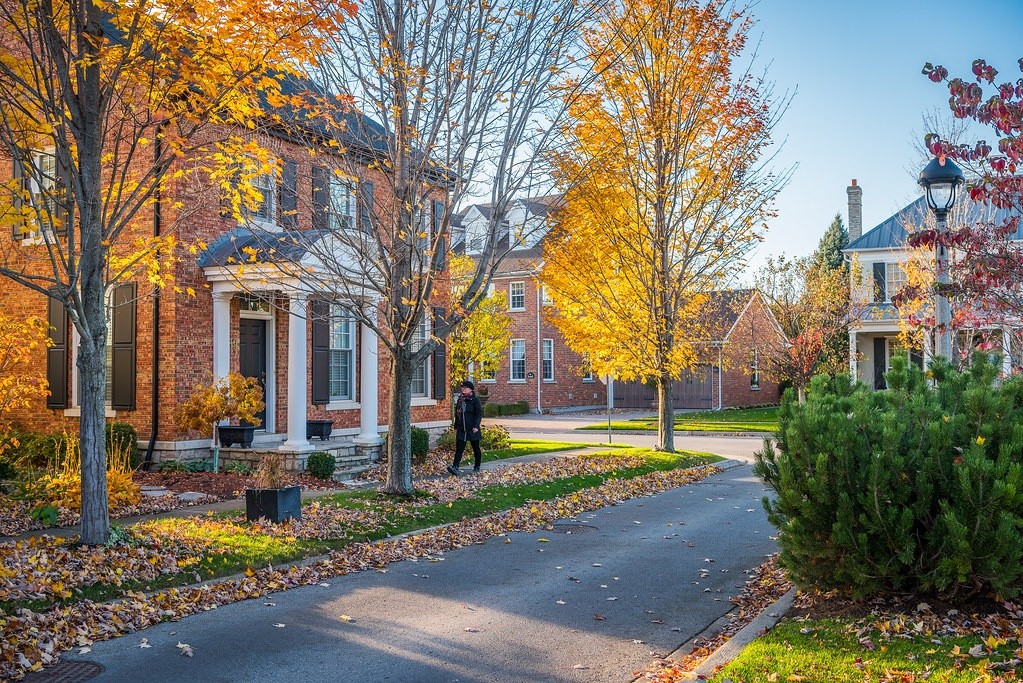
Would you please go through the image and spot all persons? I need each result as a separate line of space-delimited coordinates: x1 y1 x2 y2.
447 381 483 475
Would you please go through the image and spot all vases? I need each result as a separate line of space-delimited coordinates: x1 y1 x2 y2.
478 397 490 405
246 485 301 525
306 419 334 441
218 425 256 449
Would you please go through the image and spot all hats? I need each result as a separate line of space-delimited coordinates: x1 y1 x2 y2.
461 381 474 390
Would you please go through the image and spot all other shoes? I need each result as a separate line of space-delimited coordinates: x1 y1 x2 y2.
472 467 480 472
447 466 458 475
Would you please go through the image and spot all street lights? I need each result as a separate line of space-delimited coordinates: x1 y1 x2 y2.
916 157 965 363
